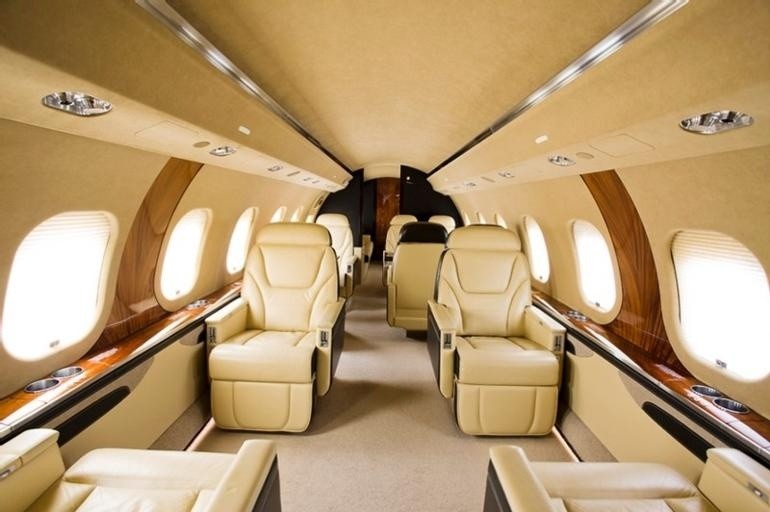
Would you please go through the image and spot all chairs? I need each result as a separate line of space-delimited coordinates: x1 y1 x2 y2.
385 221 451 343
426 222 567 437
0 426 283 512
481 442 770 511
382 213 419 274
427 215 457 235
203 210 355 433
